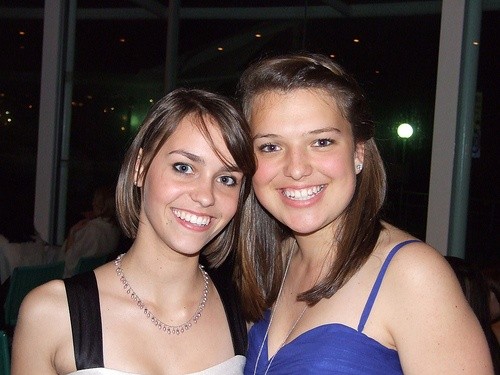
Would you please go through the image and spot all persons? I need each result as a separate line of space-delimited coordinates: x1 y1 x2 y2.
8 88 256 375
233 55 494 375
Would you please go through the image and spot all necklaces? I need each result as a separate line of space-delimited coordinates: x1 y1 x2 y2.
114 252 209 335
251 222 347 375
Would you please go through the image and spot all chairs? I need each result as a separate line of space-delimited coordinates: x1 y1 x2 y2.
0 246 125 374
